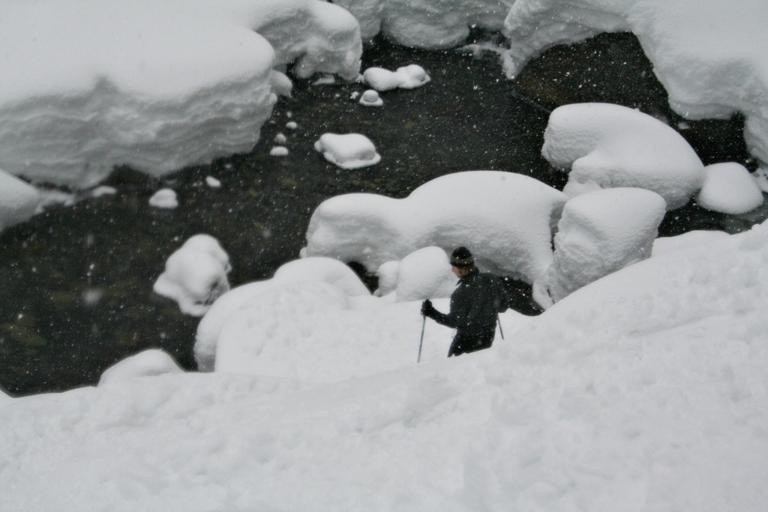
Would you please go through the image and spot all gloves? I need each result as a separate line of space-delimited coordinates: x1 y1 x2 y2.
419 300 434 319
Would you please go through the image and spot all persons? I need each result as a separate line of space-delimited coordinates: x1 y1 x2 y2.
420 246 511 357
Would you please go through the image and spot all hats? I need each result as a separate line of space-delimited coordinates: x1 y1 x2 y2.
449 246 476 267
